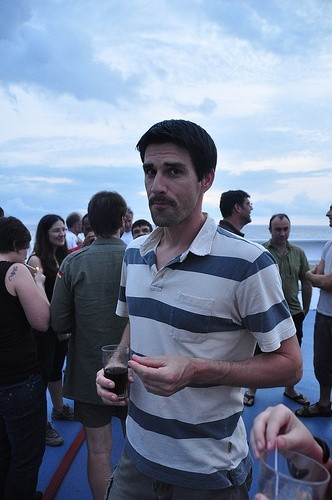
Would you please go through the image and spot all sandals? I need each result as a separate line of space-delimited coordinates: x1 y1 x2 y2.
295 401 332 417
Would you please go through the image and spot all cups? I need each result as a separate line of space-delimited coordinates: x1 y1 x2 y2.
259 449 330 500
100 345 131 400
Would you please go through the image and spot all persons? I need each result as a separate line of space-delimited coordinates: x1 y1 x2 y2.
66 213 94 255
63 212 83 250
130 219 153 239
30 213 76 448
48 190 129 500
243 213 313 407
0 216 52 500
295 203 332 419
217 189 254 238
94 119 304 500
248 403 332 500
120 207 134 246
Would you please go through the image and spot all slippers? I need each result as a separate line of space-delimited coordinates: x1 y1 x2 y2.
243 391 255 406
284 390 311 405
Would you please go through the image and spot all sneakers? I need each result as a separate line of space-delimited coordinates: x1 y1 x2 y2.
52 404 76 421
45 422 64 446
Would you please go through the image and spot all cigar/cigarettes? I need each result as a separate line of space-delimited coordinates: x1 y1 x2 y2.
23 263 36 271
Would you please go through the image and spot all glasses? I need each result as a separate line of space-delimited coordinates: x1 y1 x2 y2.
241 202 252 208
49 228 68 234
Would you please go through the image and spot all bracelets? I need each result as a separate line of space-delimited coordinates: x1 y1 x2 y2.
287 436 331 480
